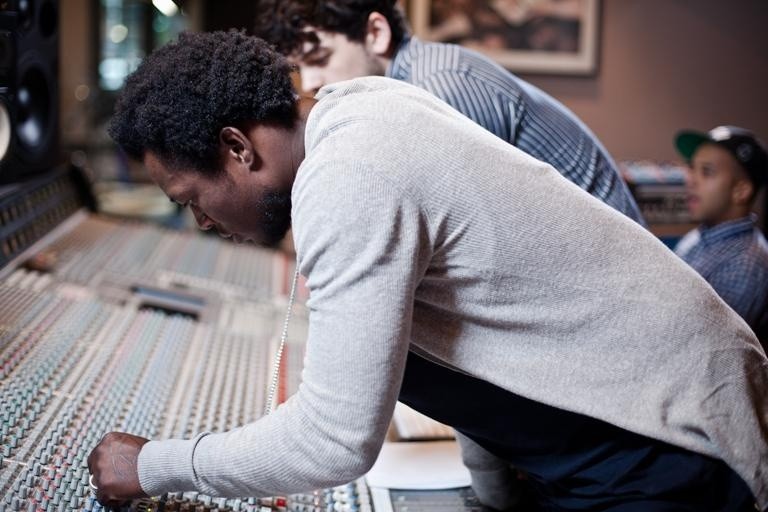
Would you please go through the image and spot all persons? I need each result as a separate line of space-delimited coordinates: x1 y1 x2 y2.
662 124 768 331
254 0 651 230
88 25 768 511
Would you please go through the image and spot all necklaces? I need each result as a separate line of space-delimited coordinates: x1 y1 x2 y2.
261 263 299 416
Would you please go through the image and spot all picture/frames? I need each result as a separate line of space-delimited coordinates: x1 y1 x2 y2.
403 1 606 78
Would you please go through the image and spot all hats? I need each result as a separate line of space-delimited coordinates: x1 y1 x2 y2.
672 125 768 190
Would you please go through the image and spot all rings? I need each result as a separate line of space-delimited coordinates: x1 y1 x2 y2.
89 472 98 495
